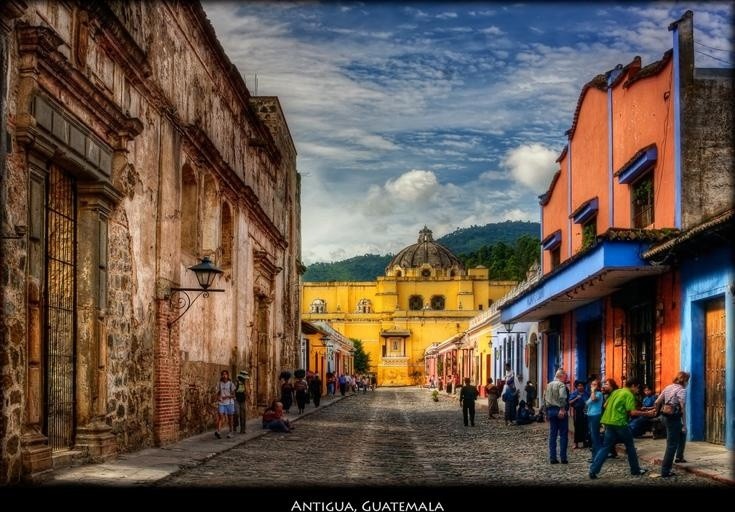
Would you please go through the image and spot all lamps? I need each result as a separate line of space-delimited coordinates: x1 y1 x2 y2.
169 255 225 325
497 323 528 334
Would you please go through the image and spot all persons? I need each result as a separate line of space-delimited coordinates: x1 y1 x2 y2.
215 368 238 440
428 363 691 477
234 370 250 435
263 370 378 434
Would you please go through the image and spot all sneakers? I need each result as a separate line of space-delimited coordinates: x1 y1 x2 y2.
590 473 597 479
662 471 675 477
227 433 235 438
675 458 687 463
550 459 568 464
609 455 620 461
633 470 647 475
215 431 222 439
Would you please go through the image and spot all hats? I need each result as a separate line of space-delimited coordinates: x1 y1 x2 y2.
238 371 250 379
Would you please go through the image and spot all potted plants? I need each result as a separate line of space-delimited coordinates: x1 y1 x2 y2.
431 389 439 402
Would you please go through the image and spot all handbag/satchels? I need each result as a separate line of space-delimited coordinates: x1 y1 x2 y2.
652 423 667 438
664 403 680 415
